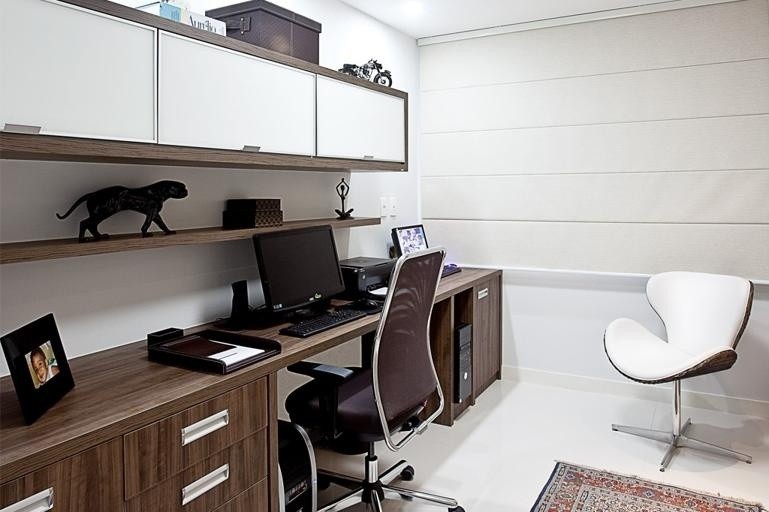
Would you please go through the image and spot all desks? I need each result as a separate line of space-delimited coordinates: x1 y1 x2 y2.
1 263 504 512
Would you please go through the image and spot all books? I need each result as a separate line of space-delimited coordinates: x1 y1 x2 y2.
168 336 238 361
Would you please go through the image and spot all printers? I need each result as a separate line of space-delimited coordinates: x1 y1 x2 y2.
332 254 396 304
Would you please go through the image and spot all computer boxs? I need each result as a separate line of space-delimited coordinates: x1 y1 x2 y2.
454 322 472 404
278 419 317 512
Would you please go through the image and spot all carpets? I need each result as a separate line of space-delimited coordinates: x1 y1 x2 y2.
530 459 767 512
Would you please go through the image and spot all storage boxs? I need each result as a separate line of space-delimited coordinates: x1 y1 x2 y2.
203 0 322 63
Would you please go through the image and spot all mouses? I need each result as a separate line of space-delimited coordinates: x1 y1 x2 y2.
449 264 457 267
360 299 379 309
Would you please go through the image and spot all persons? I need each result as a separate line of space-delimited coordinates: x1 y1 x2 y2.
30 347 60 389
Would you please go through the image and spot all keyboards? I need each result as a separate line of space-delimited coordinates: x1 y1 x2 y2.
279 307 366 336
441 266 461 278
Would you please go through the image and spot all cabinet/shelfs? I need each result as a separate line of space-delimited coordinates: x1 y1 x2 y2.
0 1 413 175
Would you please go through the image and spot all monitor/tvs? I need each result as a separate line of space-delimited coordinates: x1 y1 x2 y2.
391 224 428 257
253 224 346 323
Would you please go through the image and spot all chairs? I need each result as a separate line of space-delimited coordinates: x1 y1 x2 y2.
601 271 754 471
284 246 468 512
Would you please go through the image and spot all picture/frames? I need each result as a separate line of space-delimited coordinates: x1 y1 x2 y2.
1 312 77 426
